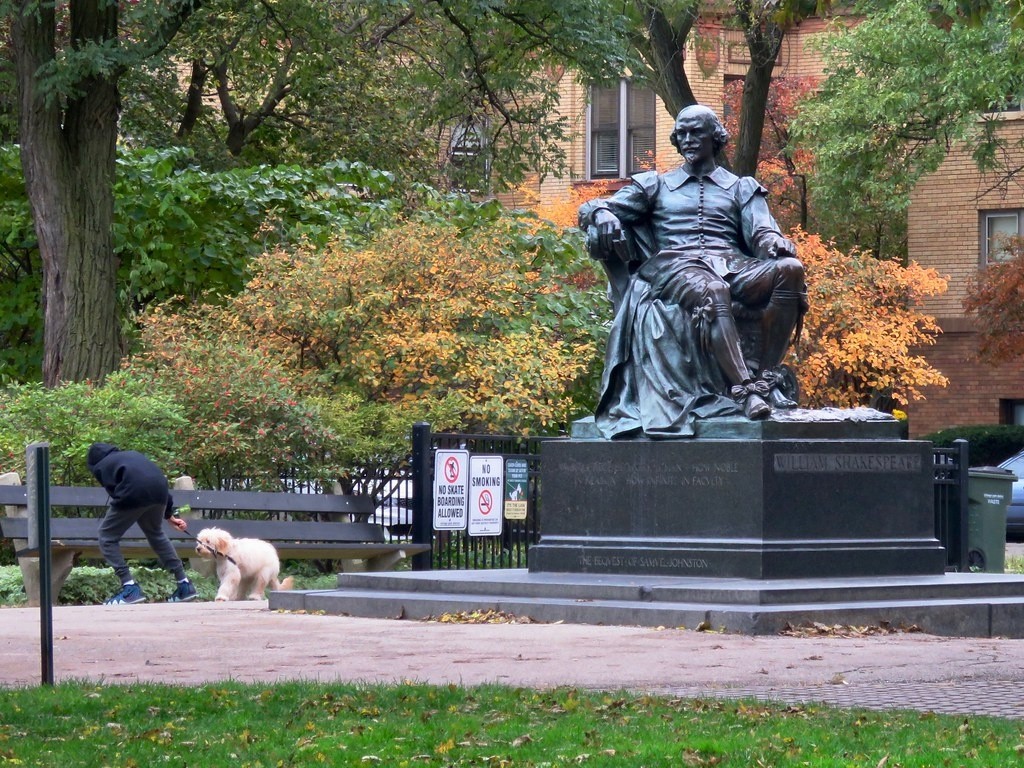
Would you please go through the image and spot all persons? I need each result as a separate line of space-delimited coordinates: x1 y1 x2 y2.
88 443 198 604
576 104 809 441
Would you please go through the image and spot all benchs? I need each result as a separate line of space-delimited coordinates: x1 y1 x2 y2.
0 479 437 610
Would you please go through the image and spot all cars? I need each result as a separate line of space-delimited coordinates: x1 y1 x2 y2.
996 449 1024 534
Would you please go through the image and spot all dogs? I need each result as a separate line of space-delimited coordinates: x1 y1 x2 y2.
195 525 294 602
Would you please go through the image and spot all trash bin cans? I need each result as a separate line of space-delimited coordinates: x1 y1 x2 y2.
965 464 1019 572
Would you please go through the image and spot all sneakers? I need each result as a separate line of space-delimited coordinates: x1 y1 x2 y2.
103 581 147 607
166 581 199 602
